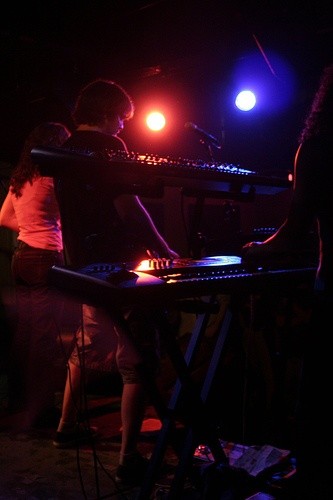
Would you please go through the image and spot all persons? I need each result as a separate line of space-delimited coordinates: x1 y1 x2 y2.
53 81 180 481
0 123 72 428
242 64 333 500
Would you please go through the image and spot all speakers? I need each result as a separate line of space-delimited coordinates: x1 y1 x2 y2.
189 204 241 256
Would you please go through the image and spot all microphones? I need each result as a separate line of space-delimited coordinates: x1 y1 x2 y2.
184 122 219 144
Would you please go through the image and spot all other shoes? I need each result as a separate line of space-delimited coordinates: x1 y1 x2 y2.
51 424 99 447
114 458 153 485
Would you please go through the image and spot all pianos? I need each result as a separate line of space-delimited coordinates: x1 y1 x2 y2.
29 151 299 449
